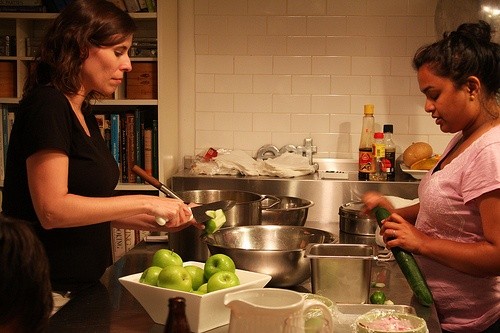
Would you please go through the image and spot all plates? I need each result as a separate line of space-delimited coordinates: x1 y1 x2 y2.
399 163 429 179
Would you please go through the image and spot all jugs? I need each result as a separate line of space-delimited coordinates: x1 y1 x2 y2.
223 288 334 333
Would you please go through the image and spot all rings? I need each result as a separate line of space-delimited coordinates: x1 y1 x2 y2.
393 230 395 237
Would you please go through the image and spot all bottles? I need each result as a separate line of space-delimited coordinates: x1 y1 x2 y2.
369 132 387 180
379 124 395 180
164 298 190 333
358 105 376 180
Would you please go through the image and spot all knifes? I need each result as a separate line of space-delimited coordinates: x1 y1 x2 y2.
156 200 236 226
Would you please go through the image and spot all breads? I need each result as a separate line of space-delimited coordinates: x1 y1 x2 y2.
403 142 442 170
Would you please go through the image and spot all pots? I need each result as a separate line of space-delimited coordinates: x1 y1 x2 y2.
339 202 376 236
165 188 281 263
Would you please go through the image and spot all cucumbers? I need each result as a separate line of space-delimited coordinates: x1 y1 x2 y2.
375 207 434 306
370 291 387 306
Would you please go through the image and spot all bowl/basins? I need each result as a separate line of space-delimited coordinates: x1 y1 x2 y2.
304 242 376 305
260 193 314 227
355 313 427 333
199 223 338 288
118 260 272 333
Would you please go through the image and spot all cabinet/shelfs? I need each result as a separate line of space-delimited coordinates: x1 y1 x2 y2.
0 0 179 236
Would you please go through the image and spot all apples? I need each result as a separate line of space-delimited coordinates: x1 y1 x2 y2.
139 249 240 295
205 209 227 234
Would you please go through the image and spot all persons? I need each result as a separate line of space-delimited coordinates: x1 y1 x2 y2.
0 0 205 316
0 215 55 333
362 24 500 333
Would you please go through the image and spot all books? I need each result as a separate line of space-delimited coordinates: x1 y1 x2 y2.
108 0 156 12
114 227 152 254
95 109 158 183
129 37 157 57
0 104 15 212
27 38 35 56
